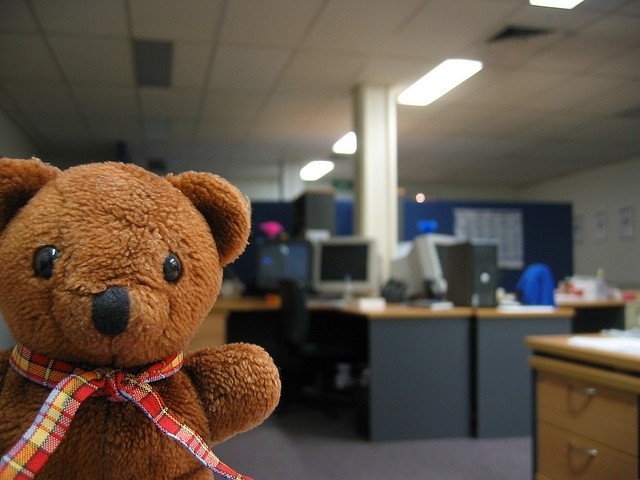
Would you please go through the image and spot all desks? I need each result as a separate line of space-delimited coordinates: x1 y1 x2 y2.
186 286 277 361
476 292 574 433
305 289 473 440
553 282 627 333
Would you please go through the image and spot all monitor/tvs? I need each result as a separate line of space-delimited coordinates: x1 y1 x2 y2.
315 239 373 297
391 234 469 299
245 237 313 297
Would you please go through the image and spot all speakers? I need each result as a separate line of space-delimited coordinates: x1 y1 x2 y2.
467 239 497 307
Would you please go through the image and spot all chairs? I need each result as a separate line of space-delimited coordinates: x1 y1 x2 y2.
277 279 361 421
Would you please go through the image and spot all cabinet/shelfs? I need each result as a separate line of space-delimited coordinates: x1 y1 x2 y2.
523 334 640 479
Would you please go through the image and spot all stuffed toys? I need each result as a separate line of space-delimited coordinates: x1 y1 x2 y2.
0 153 282 480
